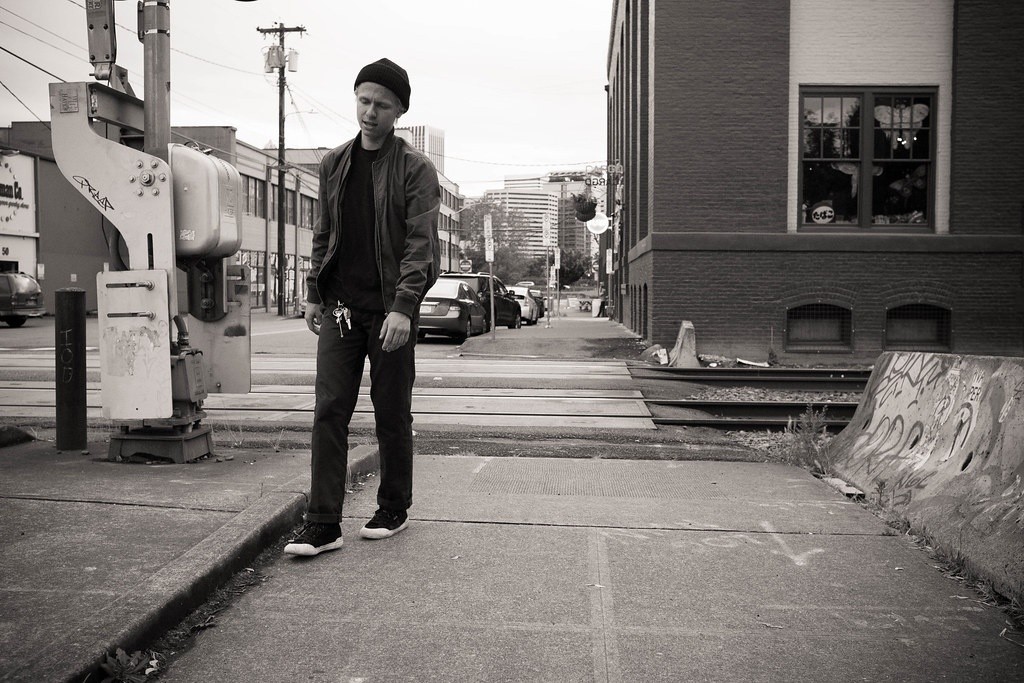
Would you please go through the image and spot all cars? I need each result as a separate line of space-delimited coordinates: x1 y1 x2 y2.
417 278 488 345
497 286 539 326
529 289 591 318
0 269 47 330
300 293 309 318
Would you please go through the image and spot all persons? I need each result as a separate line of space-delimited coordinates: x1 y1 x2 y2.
285 59 442 559
594 282 606 317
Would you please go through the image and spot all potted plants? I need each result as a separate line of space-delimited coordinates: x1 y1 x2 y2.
570 193 598 222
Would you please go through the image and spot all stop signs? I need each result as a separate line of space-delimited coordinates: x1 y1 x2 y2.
459 260 472 272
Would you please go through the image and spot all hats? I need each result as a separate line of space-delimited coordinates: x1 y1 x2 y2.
353 57 411 113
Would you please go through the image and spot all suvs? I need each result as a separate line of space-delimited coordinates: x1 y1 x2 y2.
439 270 521 334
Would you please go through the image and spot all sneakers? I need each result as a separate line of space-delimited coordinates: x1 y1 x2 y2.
361 509 410 540
282 520 344 557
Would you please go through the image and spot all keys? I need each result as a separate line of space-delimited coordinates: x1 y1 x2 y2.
331 307 352 338
